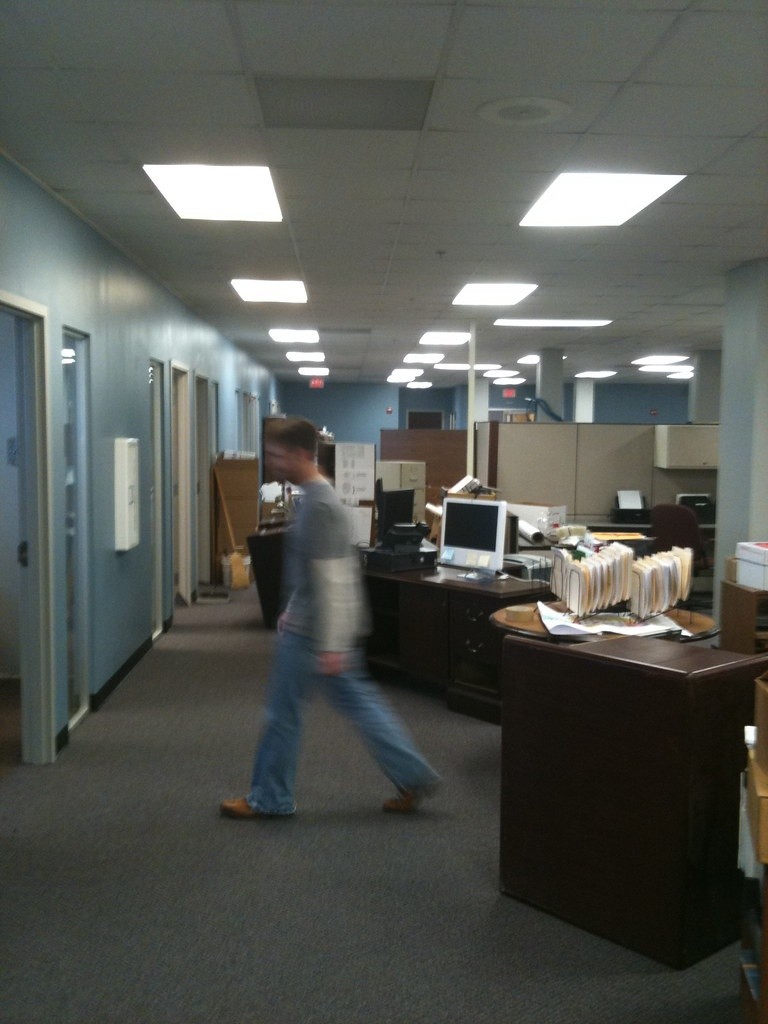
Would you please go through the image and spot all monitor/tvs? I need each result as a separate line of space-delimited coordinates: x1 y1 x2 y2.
440 498 507 571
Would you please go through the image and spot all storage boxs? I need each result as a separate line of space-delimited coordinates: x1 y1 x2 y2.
745 672 768 865
735 542 768 591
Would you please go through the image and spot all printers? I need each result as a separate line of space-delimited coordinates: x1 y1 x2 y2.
609 496 651 525
361 524 439 574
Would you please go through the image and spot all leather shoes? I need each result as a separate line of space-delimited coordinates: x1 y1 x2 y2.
221 797 296 821
382 775 447 812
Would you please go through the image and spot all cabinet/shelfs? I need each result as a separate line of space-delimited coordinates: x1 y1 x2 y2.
499 629 768 974
374 460 427 526
718 579 768 655
215 454 259 557
362 568 554 724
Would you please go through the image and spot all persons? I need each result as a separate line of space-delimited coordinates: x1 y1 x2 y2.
217 416 448 819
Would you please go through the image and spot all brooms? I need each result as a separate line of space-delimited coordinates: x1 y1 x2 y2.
213 467 251 591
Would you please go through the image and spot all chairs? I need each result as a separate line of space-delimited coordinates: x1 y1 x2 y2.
644 503 715 612
